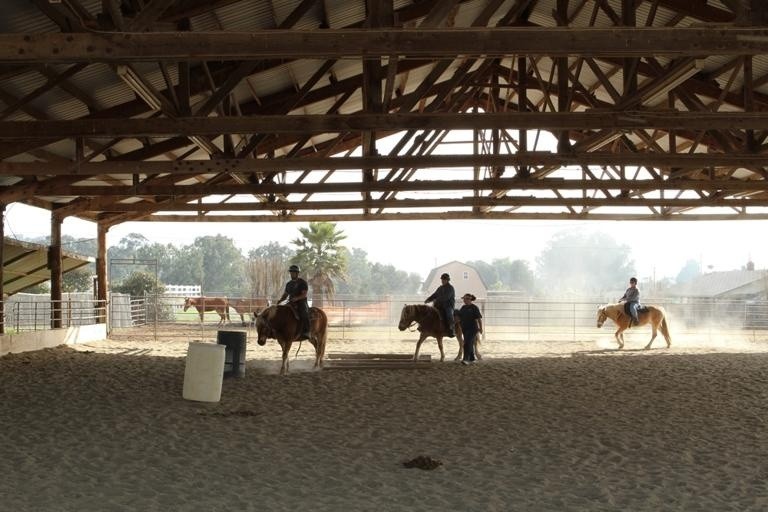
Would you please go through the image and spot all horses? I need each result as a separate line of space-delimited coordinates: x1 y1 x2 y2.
227 297 269 327
398 304 483 362
183 296 229 326
595 304 672 349
253 305 327 375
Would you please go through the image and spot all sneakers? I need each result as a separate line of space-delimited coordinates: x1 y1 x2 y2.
461 360 469 366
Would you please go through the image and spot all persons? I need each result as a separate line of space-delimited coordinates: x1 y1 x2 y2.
425 272 457 338
276 264 312 340
618 276 641 326
458 292 483 366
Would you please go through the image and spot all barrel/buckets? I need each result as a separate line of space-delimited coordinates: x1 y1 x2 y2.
217 331 247 380
182 341 226 402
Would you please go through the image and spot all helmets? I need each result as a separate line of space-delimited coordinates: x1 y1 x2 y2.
440 273 450 280
288 264 301 273
